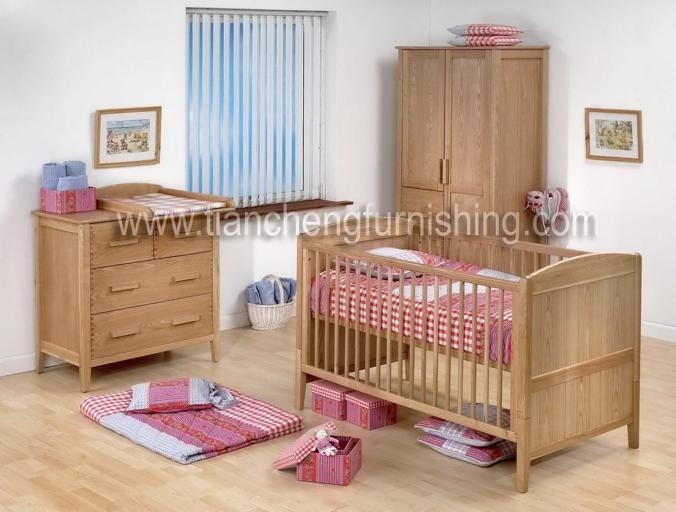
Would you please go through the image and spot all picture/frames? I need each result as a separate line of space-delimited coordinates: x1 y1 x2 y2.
93 104 162 168
583 106 644 165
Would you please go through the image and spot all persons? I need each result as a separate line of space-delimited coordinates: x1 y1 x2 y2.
107 129 117 153
134 133 148 150
119 134 128 150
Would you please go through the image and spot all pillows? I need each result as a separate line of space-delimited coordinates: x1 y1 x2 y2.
420 432 514 466
336 249 447 280
448 21 523 35
414 400 514 446
126 380 214 414
449 33 521 47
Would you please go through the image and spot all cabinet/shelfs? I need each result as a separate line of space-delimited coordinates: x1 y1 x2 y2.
33 209 227 393
391 44 550 276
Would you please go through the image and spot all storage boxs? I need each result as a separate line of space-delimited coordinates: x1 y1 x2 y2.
306 379 353 420
275 423 362 486
347 391 396 428
35 185 98 218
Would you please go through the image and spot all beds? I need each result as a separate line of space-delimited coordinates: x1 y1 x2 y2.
293 216 644 497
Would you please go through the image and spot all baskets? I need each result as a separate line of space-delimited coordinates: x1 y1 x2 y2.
246 275 294 330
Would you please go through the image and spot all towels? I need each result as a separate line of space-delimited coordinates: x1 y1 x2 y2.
57 177 90 188
64 158 86 177
42 160 64 187
244 275 296 304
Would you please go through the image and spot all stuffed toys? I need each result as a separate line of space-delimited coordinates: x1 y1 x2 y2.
311 428 339 457
